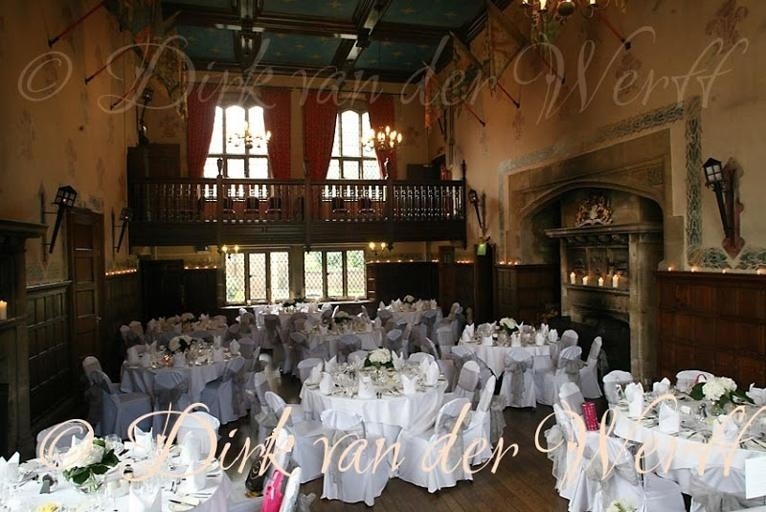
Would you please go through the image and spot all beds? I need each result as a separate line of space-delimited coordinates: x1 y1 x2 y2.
611 391 766 496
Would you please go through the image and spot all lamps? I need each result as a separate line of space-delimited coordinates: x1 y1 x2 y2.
468 189 481 228
140 87 155 140
521 0 596 25
43 185 78 254
703 158 734 238
117 206 134 252
228 121 272 147
357 124 403 153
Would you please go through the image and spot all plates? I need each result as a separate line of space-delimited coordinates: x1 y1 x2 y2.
169 496 200 512
677 430 707 445
383 389 404 397
206 458 220 473
749 440 766 451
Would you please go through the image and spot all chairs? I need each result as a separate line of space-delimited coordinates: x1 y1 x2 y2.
522 324 535 333
319 406 389 506
153 370 193 438
244 196 260 219
690 463 766 512
442 360 480 409
562 410 592 512
83 356 126 423
255 306 267 327
749 384 766 405
544 404 610 488
223 198 236 220
439 375 496 466
420 337 458 392
90 369 153 440
244 312 265 345
37 422 88 458
309 309 332 328
374 315 383 329
451 345 508 444
437 303 460 343
331 196 349 217
228 467 316 512
193 329 213 348
499 347 536 407
238 336 256 368
293 318 305 332
128 345 147 365
358 196 375 216
120 312 229 342
246 345 261 408
431 318 452 346
238 308 247 314
561 330 578 348
337 334 361 362
416 310 437 337
602 370 634 425
282 313 309 373
263 390 326 484
287 333 327 376
179 196 205 222
226 428 288 510
402 323 411 348
322 303 331 310
230 313 245 335
437 326 463 366
264 314 282 349
403 295 415 304
361 304 380 324
156 330 180 351
580 336 610 399
254 373 304 448
535 345 582 406
591 434 687 512
559 382 615 439
332 306 340 319
409 352 435 364
265 197 282 219
177 411 220 456
383 329 401 355
375 310 393 328
297 358 321 384
676 370 714 394
199 356 248 425
398 398 474 493
478 323 494 334
349 351 368 363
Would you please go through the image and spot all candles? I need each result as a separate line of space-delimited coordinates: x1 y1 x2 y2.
0 300 8 321
598 277 604 287
582 276 588 285
613 275 618 287
570 272 576 284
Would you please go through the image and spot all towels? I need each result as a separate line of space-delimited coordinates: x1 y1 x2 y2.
548 329 558 341
212 334 222 349
306 363 322 388
344 322 352 334
430 299 437 308
461 322 475 340
172 322 183 334
421 356 439 385
534 332 545 344
321 372 333 393
123 485 163 512
178 431 201 458
142 352 152 365
318 324 327 334
0 451 19 481
127 346 138 362
132 423 154 455
654 377 672 395
482 333 493 344
392 350 406 370
401 374 420 392
145 340 157 354
748 382 766 407
391 300 400 311
539 323 550 337
172 350 186 368
229 338 239 354
625 382 644 414
211 347 222 361
511 333 521 346
658 403 680 433
181 458 206 492
712 418 738 445
358 374 373 397
324 356 340 374
374 316 382 328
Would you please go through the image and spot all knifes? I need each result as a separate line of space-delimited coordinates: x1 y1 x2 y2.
752 439 766 449
169 498 197 507
